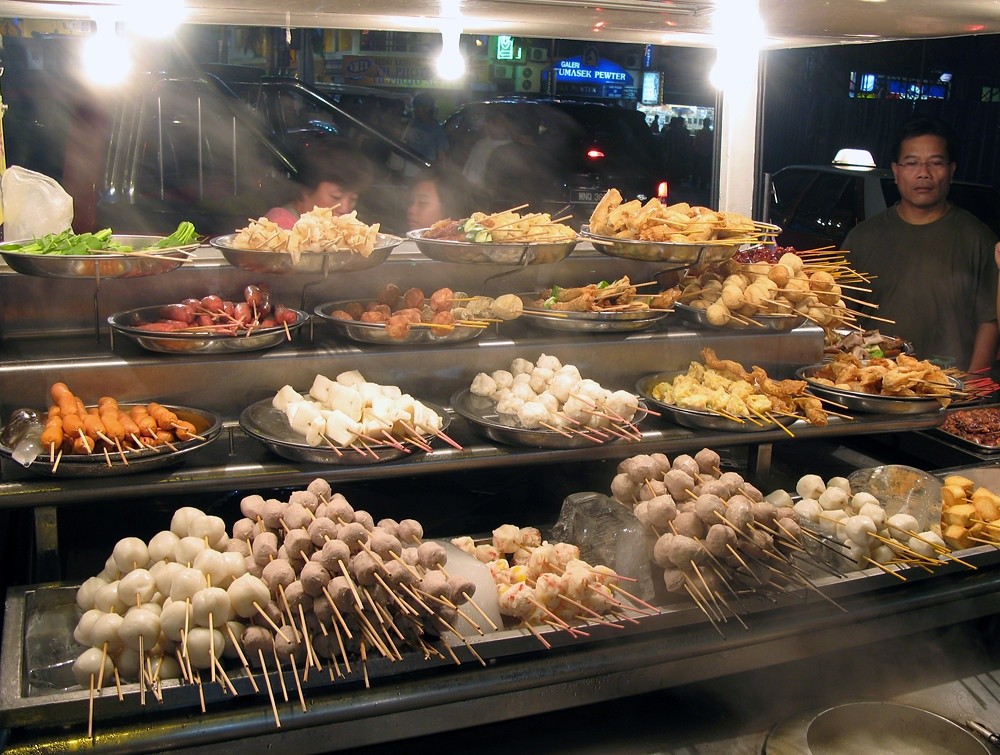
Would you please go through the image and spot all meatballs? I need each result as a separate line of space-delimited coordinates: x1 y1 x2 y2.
73 477 474 688
331 284 524 337
610 451 802 601
662 250 848 331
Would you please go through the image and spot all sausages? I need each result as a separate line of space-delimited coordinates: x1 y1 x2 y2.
39 383 197 453
127 281 296 330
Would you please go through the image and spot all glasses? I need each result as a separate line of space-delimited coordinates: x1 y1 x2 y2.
897 159 953 169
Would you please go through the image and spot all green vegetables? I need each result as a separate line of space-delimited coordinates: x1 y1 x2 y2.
0 221 201 254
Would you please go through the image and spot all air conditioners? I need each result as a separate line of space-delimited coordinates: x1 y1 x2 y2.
489 64 513 79
515 65 541 92
529 47 548 62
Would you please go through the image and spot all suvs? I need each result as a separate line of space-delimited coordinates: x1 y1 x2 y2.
769 163 1000 270
94 59 671 240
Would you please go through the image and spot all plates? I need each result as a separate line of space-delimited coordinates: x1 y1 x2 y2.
107 301 309 353
405 228 583 264
209 232 404 274
0 235 200 280
580 221 749 261
0 404 222 479
450 384 648 448
239 392 450 465
513 290 670 332
635 370 805 431
675 301 808 334
793 363 963 415
790 327 914 364
313 298 487 346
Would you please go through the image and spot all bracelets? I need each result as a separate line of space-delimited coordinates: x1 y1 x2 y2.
968 373 984 376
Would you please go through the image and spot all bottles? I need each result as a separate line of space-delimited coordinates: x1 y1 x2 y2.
640 71 665 106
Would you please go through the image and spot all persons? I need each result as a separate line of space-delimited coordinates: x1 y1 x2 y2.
405 171 478 228
647 114 713 191
0 37 113 235
264 152 362 229
335 94 588 209
827 124 1000 394
